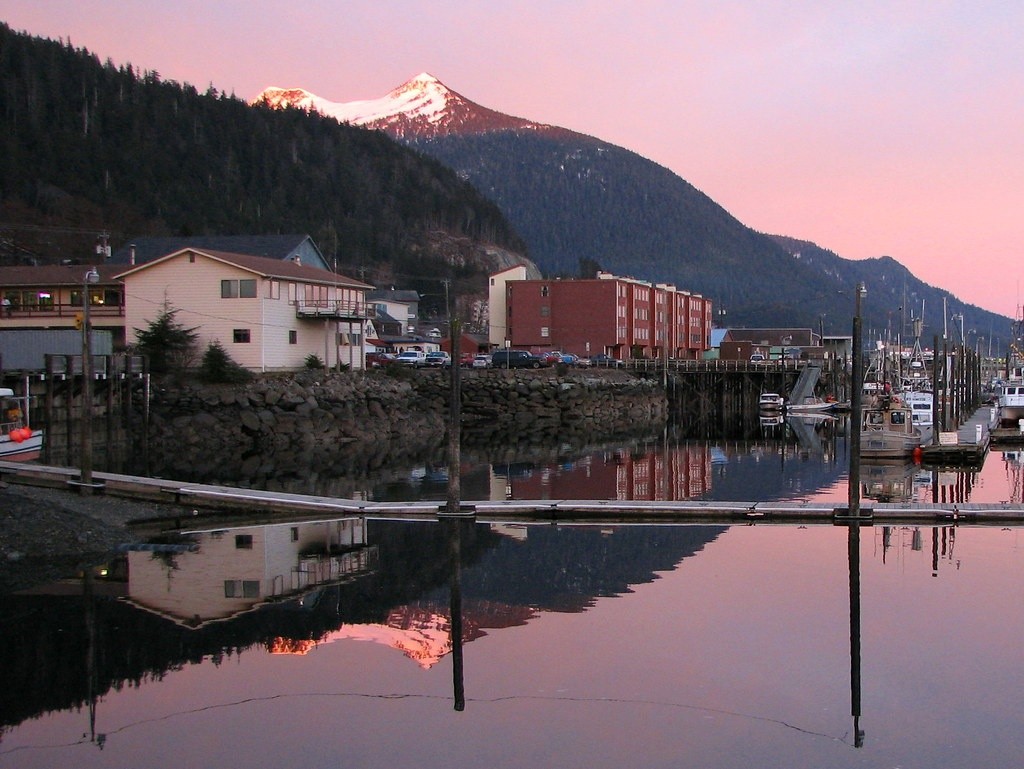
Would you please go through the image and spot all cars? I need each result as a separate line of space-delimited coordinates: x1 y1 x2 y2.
473 354 492 370
750 354 766 363
591 353 623 369
422 351 451 368
532 350 591 368
366 352 398 367
460 352 475 369
397 351 426 369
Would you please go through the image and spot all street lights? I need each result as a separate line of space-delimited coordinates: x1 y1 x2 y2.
80 265 100 489
848 282 867 515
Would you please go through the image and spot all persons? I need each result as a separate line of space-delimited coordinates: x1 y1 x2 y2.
7 402 23 422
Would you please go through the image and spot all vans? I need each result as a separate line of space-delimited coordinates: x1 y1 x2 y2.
493 350 548 369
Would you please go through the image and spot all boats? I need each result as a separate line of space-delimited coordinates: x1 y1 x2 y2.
860 457 931 565
997 336 1024 427
782 395 853 414
759 393 785 411
761 415 838 433
0 389 44 462
860 296 969 457
